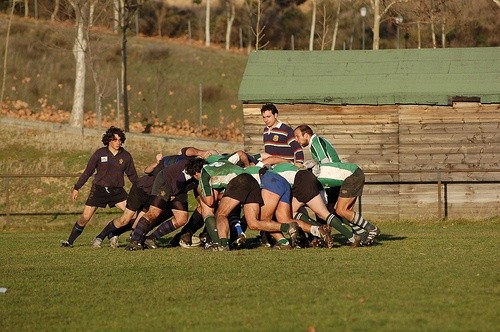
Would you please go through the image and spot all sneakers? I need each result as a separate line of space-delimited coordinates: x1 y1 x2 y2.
124 241 144 251
108 232 120 249
91 236 103 250
287 221 302 249
349 235 362 247
362 226 381 246
272 242 293 250
318 224 334 249
142 236 158 249
170 230 248 252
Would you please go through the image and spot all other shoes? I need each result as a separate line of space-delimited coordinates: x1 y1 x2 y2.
60 241 72 248
308 237 321 247
256 235 275 248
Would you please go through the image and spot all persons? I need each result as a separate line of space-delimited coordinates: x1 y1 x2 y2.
293 124 342 241
92 147 381 248
59 125 139 246
259 103 315 246
